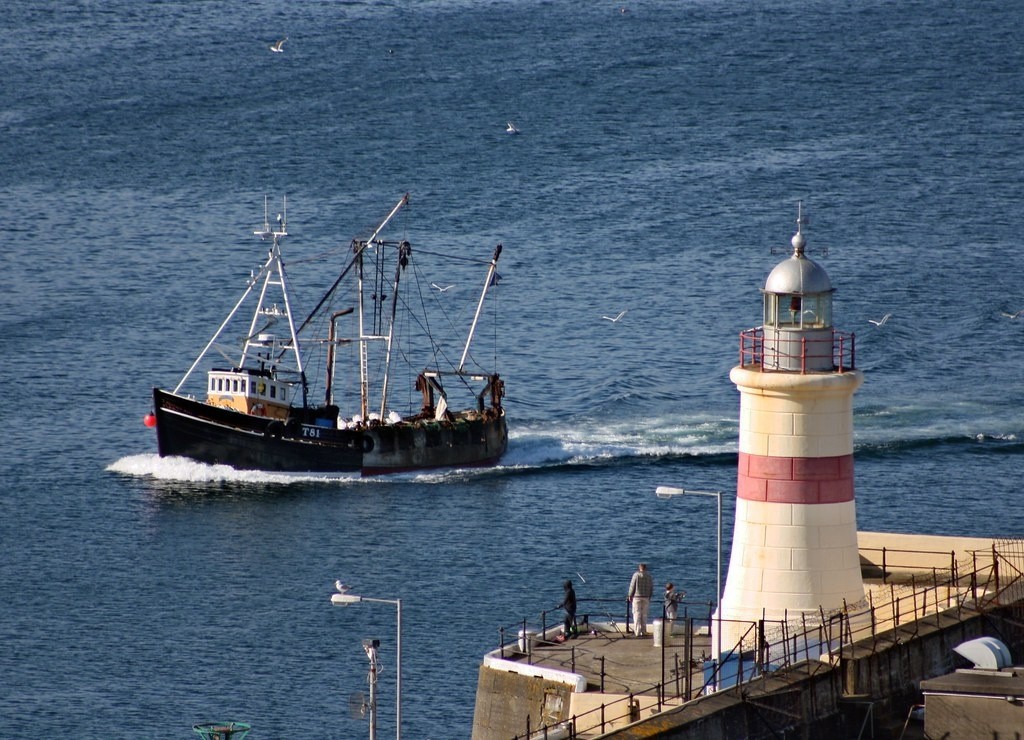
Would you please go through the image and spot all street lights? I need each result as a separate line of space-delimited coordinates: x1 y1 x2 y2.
362 638 382 740
655 485 723 693
328 593 401 740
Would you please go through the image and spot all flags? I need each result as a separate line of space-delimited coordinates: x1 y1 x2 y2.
490 272 501 286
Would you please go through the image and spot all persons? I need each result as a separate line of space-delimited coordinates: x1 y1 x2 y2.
557 580 576 638
628 563 653 637
664 582 683 637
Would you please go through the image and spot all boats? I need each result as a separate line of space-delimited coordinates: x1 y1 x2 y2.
144 188 520 473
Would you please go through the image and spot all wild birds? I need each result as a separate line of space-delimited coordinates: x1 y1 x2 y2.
1001 311 1024 320
334 580 354 595
868 314 891 327
269 37 288 53
430 281 456 293
506 123 520 134
602 311 627 323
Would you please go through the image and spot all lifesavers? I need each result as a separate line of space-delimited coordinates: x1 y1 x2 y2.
284 418 303 439
265 421 282 443
358 435 375 453
251 403 267 417
